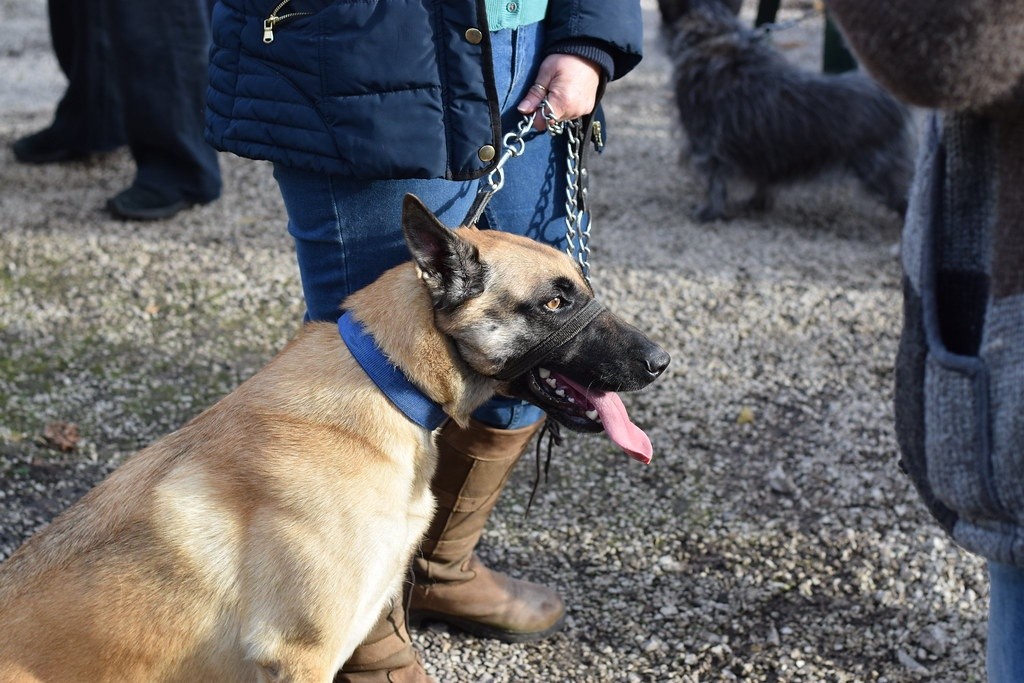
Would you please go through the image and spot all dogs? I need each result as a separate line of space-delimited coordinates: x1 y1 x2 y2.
0 191 671 683
668 0 920 224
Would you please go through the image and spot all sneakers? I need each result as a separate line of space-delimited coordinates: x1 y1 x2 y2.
107 186 193 222
11 127 88 163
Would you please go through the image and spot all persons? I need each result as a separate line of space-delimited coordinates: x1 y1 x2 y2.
12 0 221 222
202 2 642 676
824 0 1022 680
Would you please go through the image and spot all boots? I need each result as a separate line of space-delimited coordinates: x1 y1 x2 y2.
402 411 563 643
333 587 436 683
